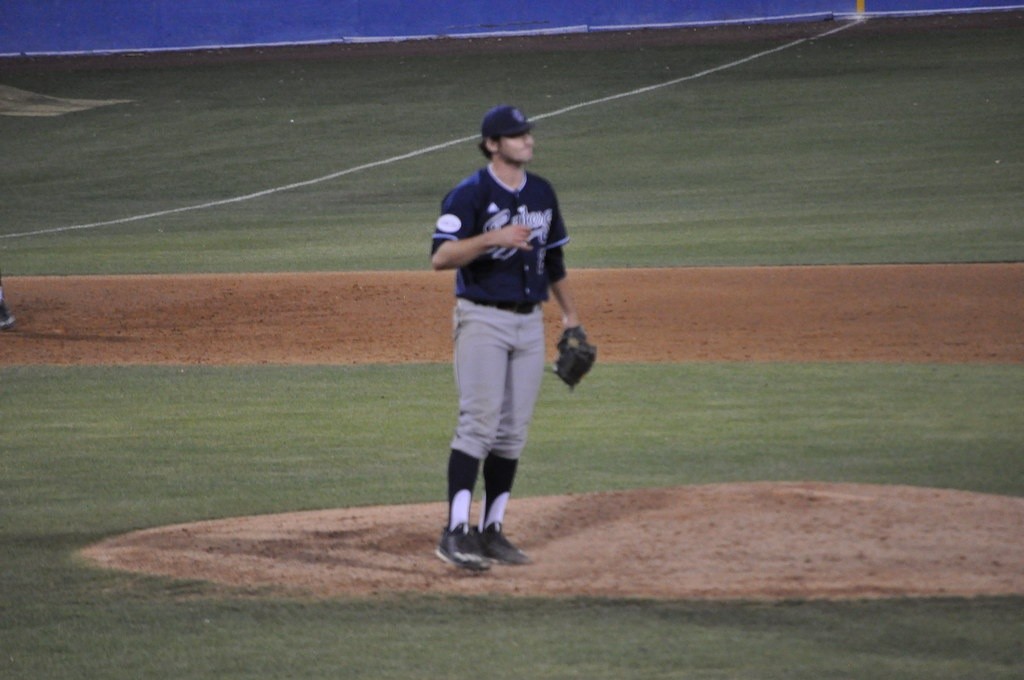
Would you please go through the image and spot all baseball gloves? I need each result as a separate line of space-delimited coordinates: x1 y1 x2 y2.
544 325 599 392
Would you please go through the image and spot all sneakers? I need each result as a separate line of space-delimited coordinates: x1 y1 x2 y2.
435 524 490 572
473 522 531 565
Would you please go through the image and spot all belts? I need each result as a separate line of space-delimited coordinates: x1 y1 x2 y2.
459 295 541 314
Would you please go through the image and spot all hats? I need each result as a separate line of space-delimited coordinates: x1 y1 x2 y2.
481 105 534 137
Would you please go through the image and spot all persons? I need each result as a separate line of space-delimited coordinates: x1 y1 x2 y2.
427 103 598 573
